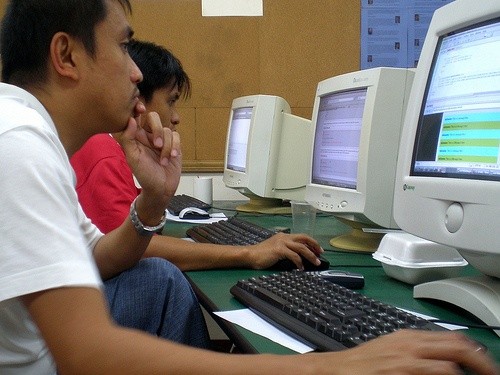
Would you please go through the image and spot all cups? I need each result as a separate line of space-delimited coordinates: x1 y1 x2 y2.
290 200 316 239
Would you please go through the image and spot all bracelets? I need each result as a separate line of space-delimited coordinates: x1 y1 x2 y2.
130 195 168 234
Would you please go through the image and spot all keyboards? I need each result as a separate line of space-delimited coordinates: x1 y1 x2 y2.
188 216 281 250
231 268 452 355
167 194 212 217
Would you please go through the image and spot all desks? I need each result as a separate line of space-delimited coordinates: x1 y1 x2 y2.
161 201 500 375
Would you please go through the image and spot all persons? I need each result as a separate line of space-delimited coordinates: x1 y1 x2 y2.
70 39 324 271
0 0 500 375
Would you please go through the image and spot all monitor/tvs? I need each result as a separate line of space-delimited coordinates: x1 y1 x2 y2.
224 93 297 213
391 0 500 340
245 96 320 216
300 68 416 255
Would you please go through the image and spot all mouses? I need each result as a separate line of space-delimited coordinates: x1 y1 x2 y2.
269 252 329 271
178 206 211 219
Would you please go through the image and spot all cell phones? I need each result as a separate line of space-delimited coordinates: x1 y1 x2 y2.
305 269 363 290
